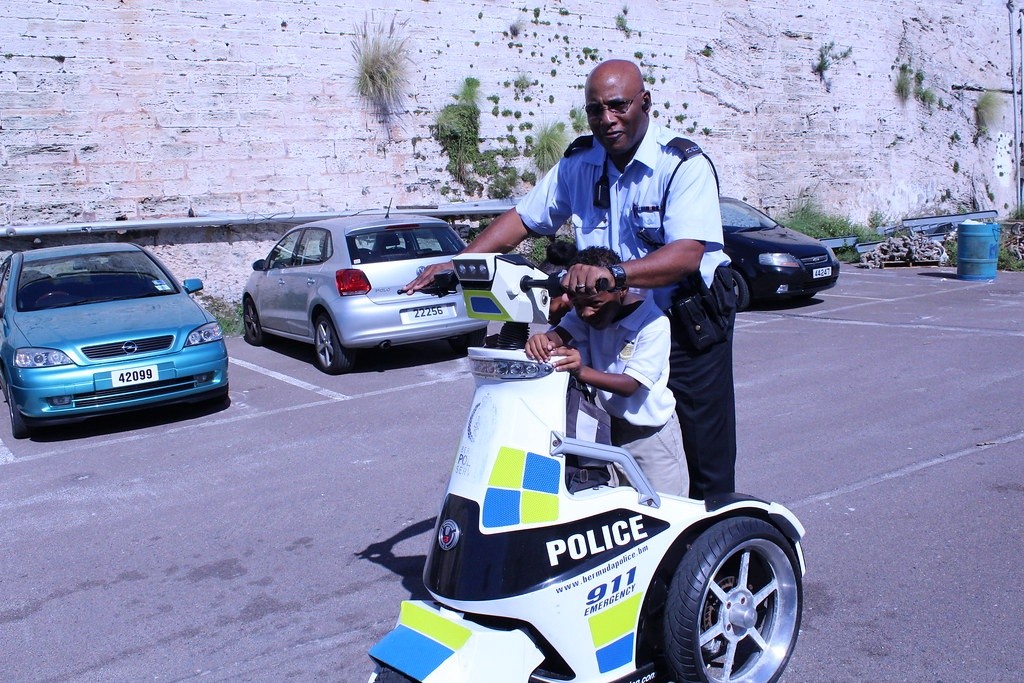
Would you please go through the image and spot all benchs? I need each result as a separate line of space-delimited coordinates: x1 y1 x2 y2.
18 271 167 308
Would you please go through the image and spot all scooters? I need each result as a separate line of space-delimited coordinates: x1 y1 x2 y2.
362 249 809 683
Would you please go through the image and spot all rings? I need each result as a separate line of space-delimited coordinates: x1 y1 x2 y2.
576 284 585 288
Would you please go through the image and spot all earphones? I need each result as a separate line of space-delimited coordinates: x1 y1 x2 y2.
641 97 650 111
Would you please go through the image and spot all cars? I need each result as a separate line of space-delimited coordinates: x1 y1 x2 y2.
0 242 229 438
242 214 490 376
719 196 840 313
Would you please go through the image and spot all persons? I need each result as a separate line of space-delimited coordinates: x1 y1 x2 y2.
525 245 690 497
402 58 738 500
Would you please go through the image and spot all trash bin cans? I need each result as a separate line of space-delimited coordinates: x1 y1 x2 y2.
957 222 1000 280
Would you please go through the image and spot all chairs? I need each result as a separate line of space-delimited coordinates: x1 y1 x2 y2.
372 232 407 257
351 248 378 265
346 236 361 260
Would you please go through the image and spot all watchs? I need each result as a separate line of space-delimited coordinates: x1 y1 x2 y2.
606 265 626 293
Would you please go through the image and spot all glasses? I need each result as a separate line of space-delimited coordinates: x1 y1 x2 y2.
585 88 643 117
